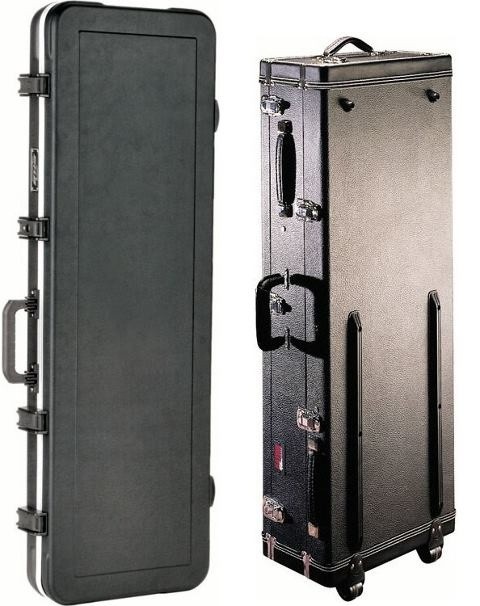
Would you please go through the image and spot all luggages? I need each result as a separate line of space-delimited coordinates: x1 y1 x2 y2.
3 0 220 605
253 35 455 600
